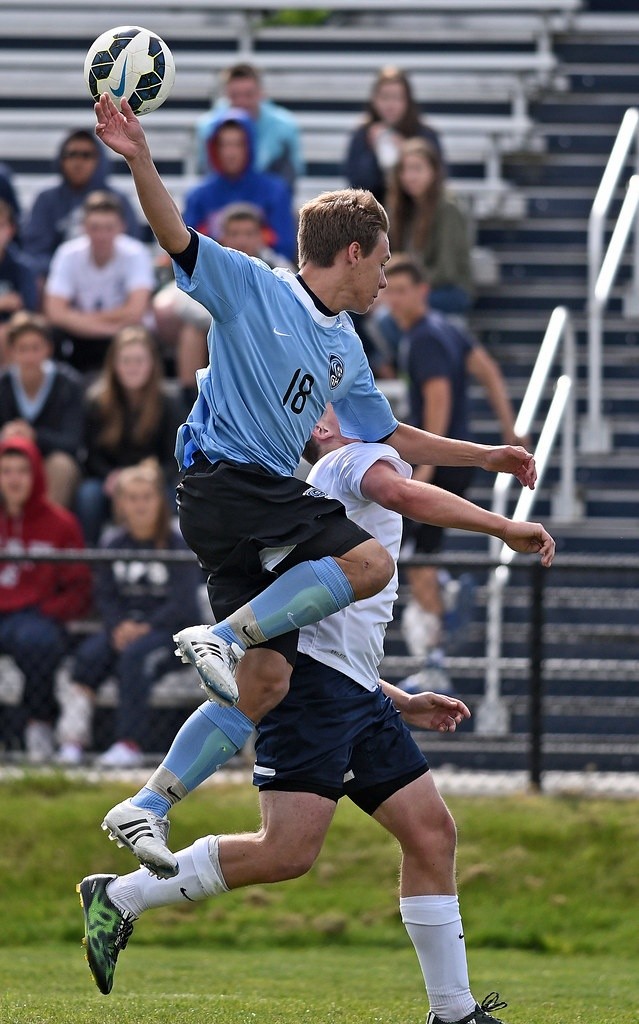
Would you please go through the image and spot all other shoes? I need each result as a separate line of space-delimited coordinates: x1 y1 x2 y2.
3 700 147 771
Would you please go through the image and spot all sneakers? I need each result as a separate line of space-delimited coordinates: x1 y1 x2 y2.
426 1002 505 1024
76 873 134 994
100 797 179 880
171 623 245 709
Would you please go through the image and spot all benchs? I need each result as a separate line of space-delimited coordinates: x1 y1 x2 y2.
2 0 581 708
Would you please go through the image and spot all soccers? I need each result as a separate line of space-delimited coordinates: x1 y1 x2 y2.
83 25 177 117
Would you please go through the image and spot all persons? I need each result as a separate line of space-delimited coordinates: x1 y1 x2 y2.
95 92 537 883
0 60 531 771
77 393 558 1024
